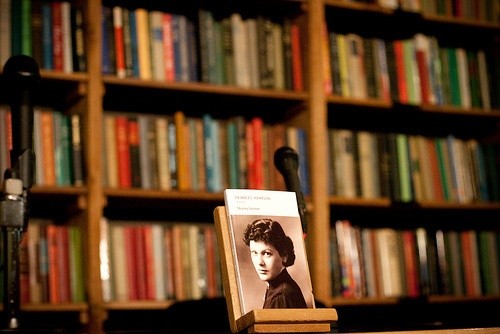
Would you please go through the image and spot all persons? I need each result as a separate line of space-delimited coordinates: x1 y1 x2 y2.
242 218 307 310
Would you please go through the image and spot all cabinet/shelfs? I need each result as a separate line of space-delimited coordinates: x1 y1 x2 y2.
1 1 500 334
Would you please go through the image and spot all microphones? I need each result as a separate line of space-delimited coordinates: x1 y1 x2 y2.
1 54 41 240
274 146 308 234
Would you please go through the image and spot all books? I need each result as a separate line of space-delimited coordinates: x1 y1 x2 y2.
223 189 317 316
10 0 500 304
